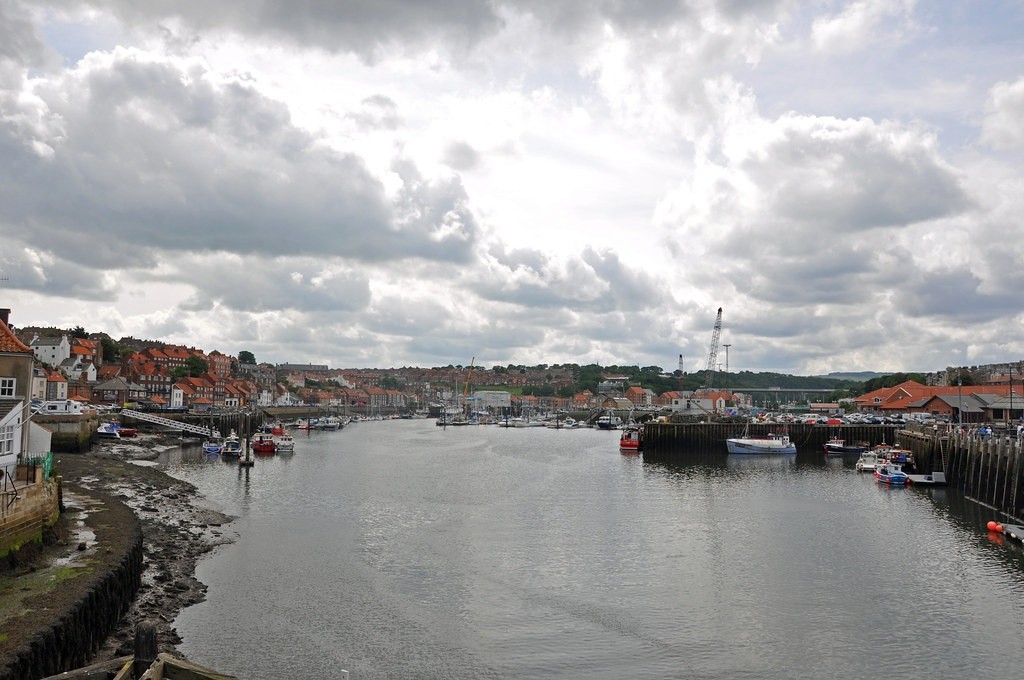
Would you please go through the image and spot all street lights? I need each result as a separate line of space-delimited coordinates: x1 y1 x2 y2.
958 379 963 429
1009 362 1013 428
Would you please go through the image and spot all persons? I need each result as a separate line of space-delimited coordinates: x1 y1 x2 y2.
956 423 1024 447
933 423 937 436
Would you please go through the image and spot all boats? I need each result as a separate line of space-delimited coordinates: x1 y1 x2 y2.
856 445 946 485
726 436 797 454
436 410 622 428
98 421 137 440
252 433 275 454
823 440 868 456
620 427 643 452
997 520 1024 545
262 413 399 436
276 436 295 454
203 428 243 457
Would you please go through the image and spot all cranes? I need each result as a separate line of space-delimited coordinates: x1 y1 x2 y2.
462 356 477 405
705 307 724 389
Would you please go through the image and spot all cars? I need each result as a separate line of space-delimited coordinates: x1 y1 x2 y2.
747 413 953 425
30 398 45 416
620 403 672 412
88 401 190 413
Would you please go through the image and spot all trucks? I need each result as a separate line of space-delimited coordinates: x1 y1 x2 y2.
46 400 90 414
